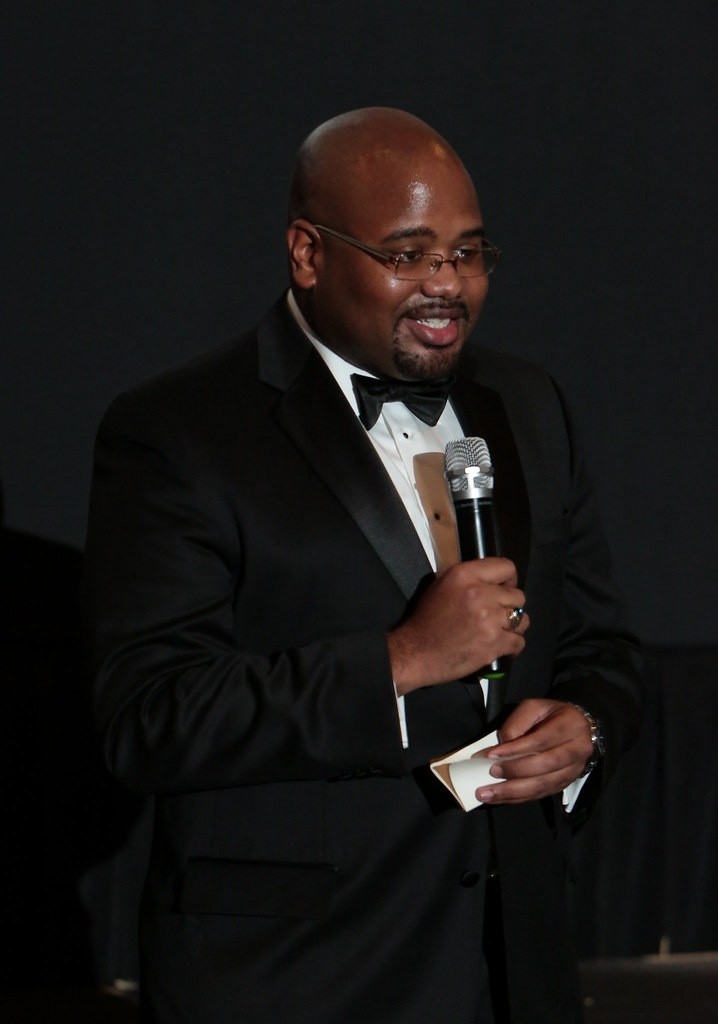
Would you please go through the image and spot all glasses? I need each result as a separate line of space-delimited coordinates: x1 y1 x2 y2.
315 224 502 282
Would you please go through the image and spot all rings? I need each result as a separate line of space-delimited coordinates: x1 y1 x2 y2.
506 606 524 631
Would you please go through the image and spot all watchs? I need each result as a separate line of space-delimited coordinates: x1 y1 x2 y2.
570 702 605 778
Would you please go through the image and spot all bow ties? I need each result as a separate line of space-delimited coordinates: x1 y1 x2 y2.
350 375 456 430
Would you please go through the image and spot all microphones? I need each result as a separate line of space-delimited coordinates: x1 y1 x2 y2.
445 436 505 680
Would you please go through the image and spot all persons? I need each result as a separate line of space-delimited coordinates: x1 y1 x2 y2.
84 106 646 1024
